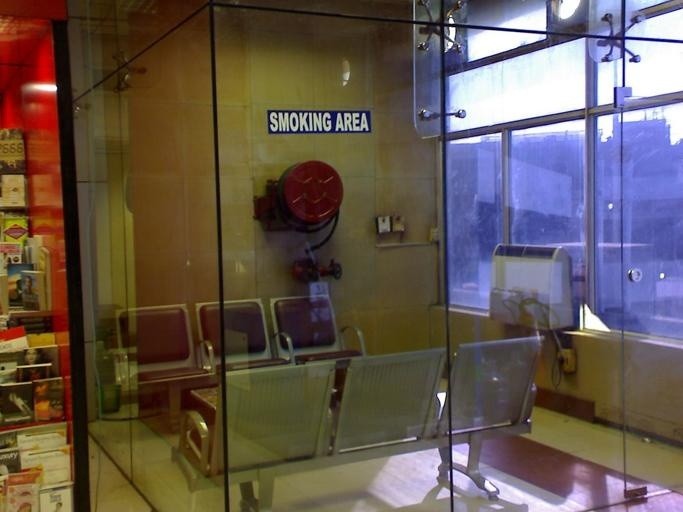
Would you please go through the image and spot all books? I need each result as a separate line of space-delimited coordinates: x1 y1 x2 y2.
0 128 75 512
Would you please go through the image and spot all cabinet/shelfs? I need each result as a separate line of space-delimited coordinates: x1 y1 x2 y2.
0 0 92 510
